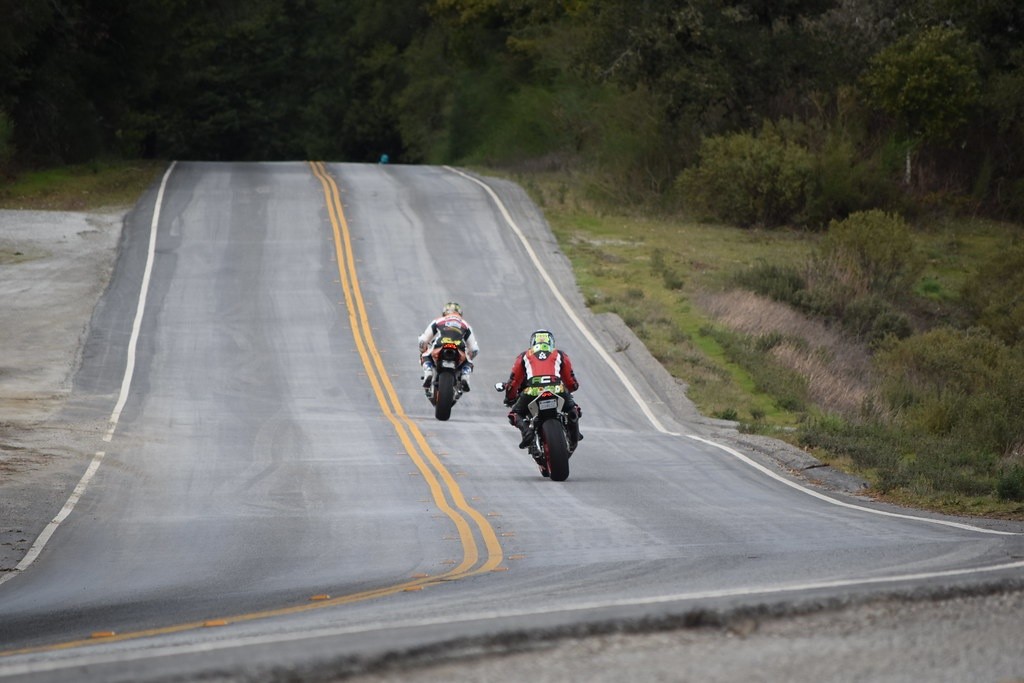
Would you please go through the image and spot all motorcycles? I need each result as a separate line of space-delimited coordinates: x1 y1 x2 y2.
494 380 584 482
422 342 467 421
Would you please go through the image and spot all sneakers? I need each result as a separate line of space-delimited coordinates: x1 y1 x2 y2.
577 433 582 441
423 361 433 388
518 430 536 449
461 365 471 392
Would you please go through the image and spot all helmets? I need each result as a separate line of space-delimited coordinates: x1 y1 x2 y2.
442 300 463 318
529 330 555 353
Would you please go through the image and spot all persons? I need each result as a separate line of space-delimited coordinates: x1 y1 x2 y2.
419 302 478 392
505 329 584 448
